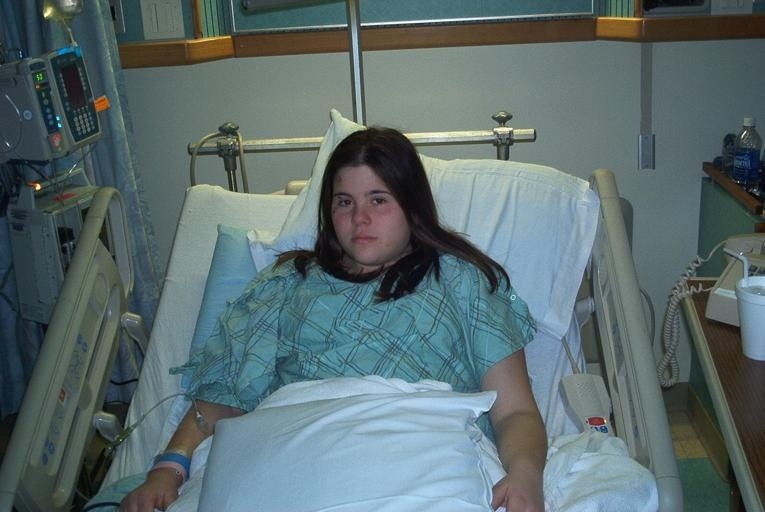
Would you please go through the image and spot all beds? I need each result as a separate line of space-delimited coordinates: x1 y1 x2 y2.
1 168 686 510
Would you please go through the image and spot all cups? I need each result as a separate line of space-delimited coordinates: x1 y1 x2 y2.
735 276 765 362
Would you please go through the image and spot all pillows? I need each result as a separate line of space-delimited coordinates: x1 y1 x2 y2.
249 109 602 342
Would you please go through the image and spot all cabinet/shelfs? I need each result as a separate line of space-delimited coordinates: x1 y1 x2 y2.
681 160 764 484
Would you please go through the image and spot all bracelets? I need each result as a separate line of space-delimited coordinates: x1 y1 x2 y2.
148 448 189 483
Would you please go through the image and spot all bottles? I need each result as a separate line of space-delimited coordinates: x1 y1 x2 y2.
733 117 763 193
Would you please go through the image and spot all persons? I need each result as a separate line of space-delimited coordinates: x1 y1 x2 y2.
119 127 547 512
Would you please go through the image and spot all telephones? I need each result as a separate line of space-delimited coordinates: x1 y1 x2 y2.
705 233 765 327
